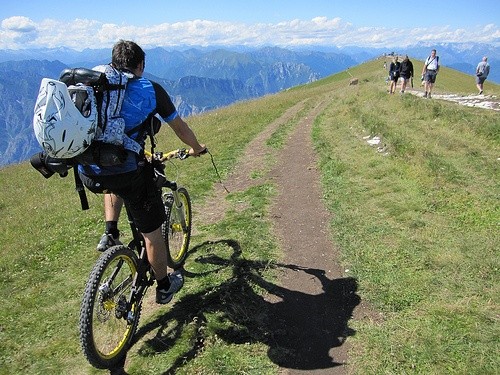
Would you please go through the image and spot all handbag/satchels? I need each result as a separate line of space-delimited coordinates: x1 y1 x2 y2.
476 72 482 76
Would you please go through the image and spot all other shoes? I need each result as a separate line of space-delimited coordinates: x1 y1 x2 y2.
478 90 483 94
423 92 431 98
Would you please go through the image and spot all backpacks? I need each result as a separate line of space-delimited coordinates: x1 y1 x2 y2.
92 64 143 155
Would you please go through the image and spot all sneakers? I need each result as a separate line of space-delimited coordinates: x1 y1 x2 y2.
155 270 184 305
97 233 124 251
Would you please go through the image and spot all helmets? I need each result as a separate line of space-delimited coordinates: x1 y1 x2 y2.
32 78 98 159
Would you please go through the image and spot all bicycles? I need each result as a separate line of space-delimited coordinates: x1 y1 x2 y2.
79 147 209 370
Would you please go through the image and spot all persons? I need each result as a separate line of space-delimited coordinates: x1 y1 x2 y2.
399 55 414 93
77 40 206 304
389 56 401 95
476 56 490 94
421 50 440 99
